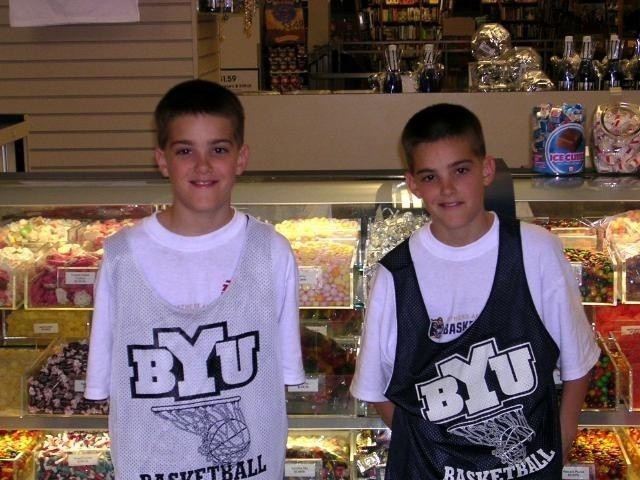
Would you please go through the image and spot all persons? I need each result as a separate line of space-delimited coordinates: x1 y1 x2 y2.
346 104 601 480
82 79 305 480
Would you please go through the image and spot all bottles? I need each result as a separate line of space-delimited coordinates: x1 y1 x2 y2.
418 43 443 93
382 43 404 93
591 102 639 177
601 34 624 92
576 35 601 92
631 38 640 89
557 35 576 91
197 0 232 14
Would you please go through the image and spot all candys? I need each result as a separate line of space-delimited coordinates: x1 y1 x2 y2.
530 101 586 177
275 212 430 480
590 103 640 176
1 216 141 480
531 210 640 480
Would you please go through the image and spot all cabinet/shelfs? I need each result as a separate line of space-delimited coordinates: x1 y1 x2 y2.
506 163 640 480
0 158 545 480
